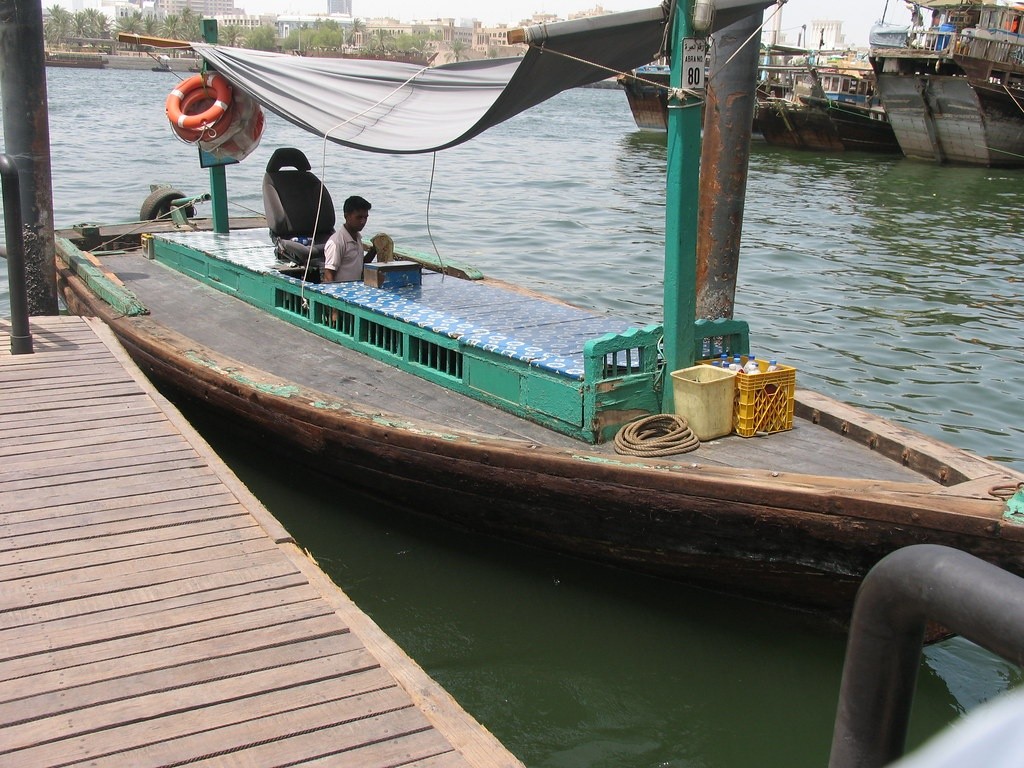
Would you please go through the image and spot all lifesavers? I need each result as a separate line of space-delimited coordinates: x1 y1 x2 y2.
165 73 265 153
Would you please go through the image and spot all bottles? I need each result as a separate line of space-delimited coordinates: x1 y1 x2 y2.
712 354 780 375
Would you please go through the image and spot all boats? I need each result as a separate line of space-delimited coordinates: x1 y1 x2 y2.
46 1 1024 657
613 0 1024 169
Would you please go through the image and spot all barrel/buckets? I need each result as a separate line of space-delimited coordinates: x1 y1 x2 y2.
935 23 957 50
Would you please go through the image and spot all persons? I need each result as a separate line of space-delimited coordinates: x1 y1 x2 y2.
324 196 378 327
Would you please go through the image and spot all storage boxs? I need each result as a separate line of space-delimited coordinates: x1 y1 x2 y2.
364 261 424 289
695 354 797 437
